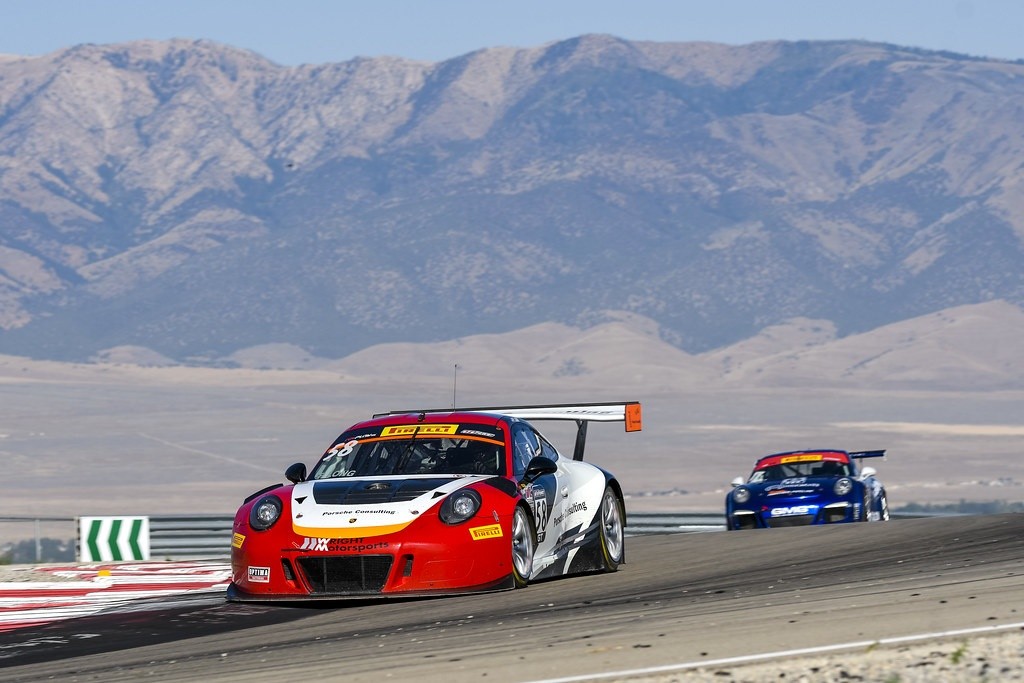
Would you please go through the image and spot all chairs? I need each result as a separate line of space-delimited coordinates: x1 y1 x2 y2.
438 447 480 474
765 467 788 481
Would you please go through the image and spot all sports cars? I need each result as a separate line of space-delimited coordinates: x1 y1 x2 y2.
724 451 891 530
228 400 642 603
